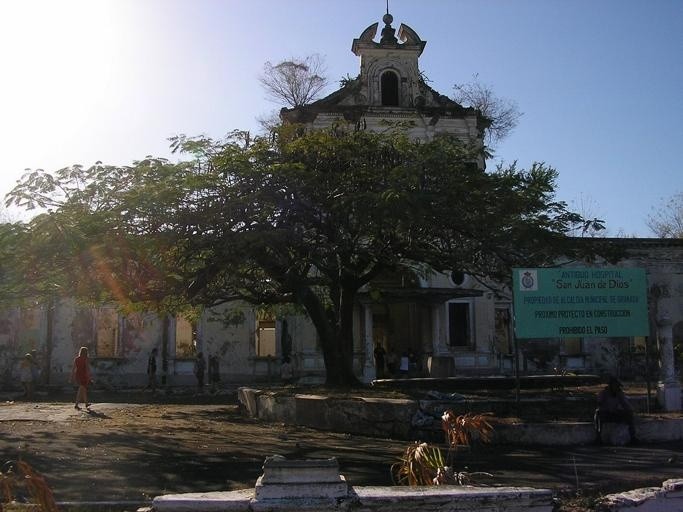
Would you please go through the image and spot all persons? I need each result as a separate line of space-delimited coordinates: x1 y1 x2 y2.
208 355 220 394
193 352 206 388
374 342 387 380
20 353 34 399
593 378 639 447
280 357 292 386
68 346 93 410
386 347 397 376
406 348 418 369
146 349 158 391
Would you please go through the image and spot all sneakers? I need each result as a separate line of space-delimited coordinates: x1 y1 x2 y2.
74 404 92 410
